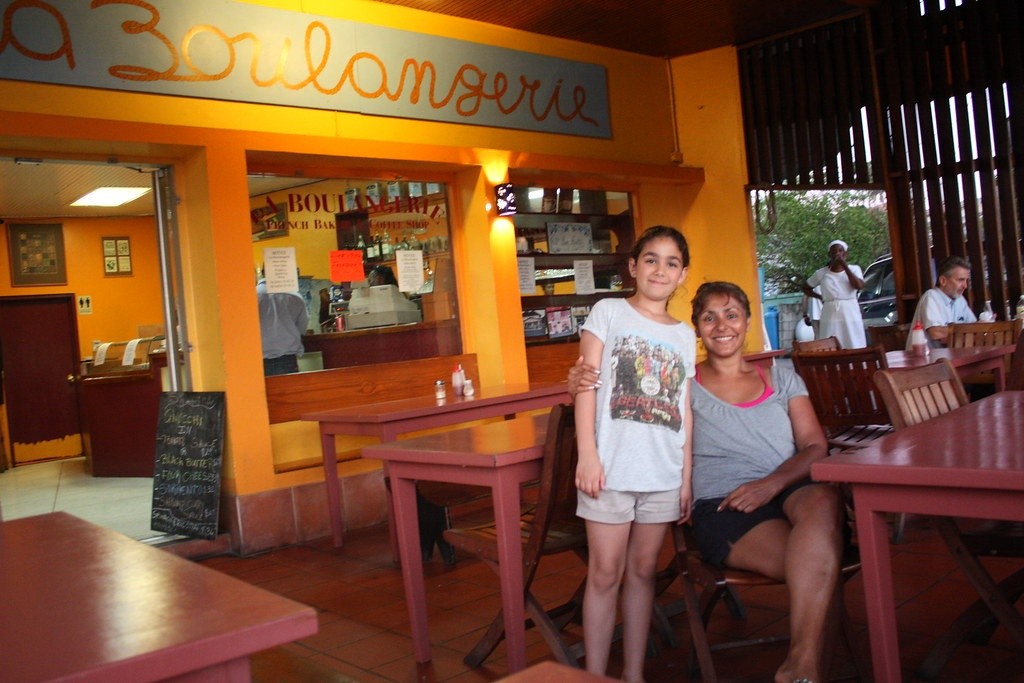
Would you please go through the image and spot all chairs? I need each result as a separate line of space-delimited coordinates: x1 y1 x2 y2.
463 316 1024 683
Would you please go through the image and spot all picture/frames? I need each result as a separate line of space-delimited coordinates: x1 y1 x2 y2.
6 221 68 287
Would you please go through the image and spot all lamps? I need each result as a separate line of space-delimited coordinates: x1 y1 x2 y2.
494 182 518 217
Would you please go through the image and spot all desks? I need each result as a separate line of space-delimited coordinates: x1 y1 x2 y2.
743 348 787 367
299 384 571 563
360 413 577 675
810 392 1024 683
0 510 321 683
825 343 1015 391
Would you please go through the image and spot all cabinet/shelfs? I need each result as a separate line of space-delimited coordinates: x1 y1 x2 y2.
515 206 637 346
333 192 451 268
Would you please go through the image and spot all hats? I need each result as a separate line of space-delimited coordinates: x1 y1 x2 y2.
827 240 849 252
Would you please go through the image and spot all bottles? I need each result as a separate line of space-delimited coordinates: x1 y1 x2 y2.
355 231 451 264
434 379 446 399
911 320 927 357
451 363 475 397
983 300 996 322
1016 295 1024 329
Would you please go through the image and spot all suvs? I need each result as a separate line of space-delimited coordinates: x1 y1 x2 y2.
793 245 935 343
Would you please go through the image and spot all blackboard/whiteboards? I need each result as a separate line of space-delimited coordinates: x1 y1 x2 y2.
149 390 224 542
545 222 594 253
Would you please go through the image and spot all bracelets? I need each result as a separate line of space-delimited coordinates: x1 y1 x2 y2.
803 315 807 317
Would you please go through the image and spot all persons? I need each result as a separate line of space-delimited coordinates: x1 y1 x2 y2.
567 281 846 683
256 265 310 376
803 285 821 340
802 240 867 350
575 226 696 683
907 258 997 350
